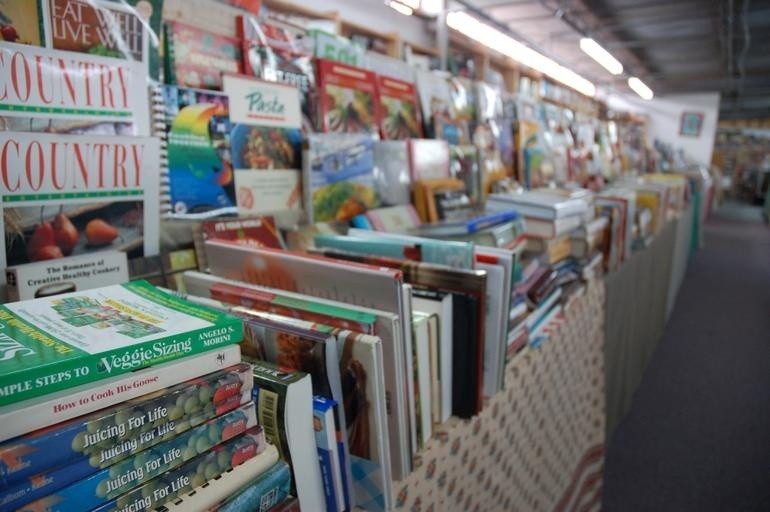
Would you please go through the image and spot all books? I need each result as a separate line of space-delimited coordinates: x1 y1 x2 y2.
0 1 529 194
525 75 700 339
1 196 528 511
